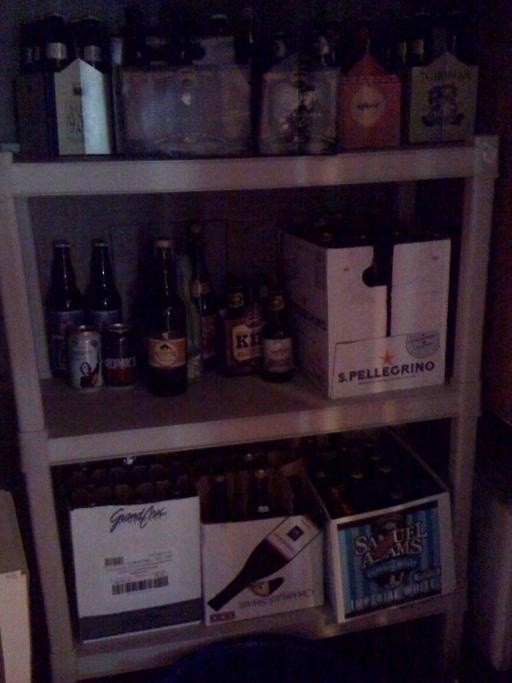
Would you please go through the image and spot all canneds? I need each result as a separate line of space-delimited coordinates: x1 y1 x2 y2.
104 323 138 386
67 324 103 391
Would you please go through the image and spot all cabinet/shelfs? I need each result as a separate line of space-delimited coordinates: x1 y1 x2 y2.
0 133 499 682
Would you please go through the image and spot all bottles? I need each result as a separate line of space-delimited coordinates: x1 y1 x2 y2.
205 517 325 611
257 294 298 382
18 9 468 151
219 289 256 377
85 238 124 333
185 216 222 368
66 436 438 513
43 241 90 377
310 224 447 284
145 239 189 399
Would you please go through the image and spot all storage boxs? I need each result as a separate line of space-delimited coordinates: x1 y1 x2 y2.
200 452 326 626
453 479 510 683
57 465 201 643
277 224 451 399
0 490 35 680
279 427 458 624
